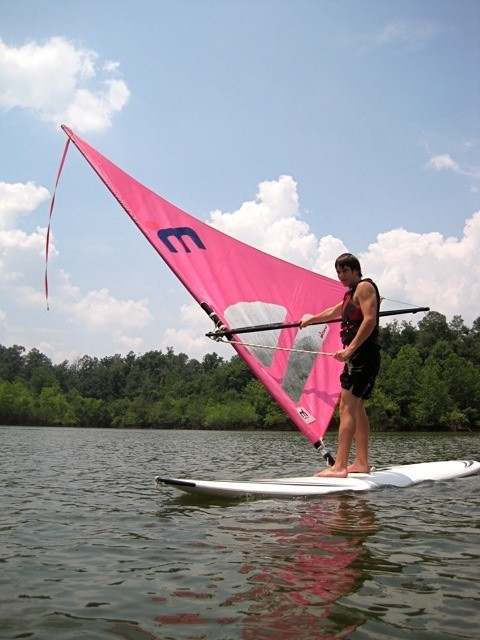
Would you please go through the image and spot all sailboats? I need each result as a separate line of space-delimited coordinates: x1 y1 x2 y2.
44 123 480 499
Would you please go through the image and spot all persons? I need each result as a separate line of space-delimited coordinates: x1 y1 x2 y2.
298 252 382 480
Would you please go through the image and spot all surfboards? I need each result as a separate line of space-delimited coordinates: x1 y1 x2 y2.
153 459 480 499
